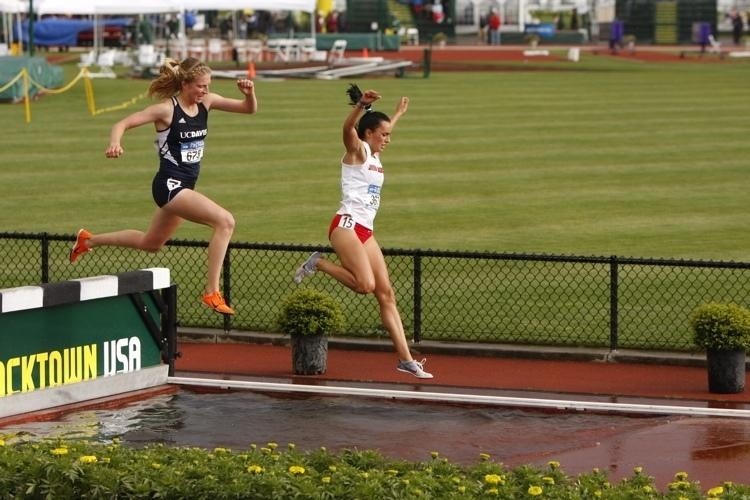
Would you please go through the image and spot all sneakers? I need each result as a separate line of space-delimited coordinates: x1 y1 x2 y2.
395 356 434 380
199 285 236 316
291 250 321 284
68 227 95 265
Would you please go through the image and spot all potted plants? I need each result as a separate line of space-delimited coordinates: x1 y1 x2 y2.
277 288 345 376
690 300 750 394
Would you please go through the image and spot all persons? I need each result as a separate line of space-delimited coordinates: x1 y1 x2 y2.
69 56 257 315
294 81 433 379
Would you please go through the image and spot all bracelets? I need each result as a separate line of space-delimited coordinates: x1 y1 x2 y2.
356 100 366 109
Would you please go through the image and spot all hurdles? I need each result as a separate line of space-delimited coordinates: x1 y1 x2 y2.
0 267 181 418
